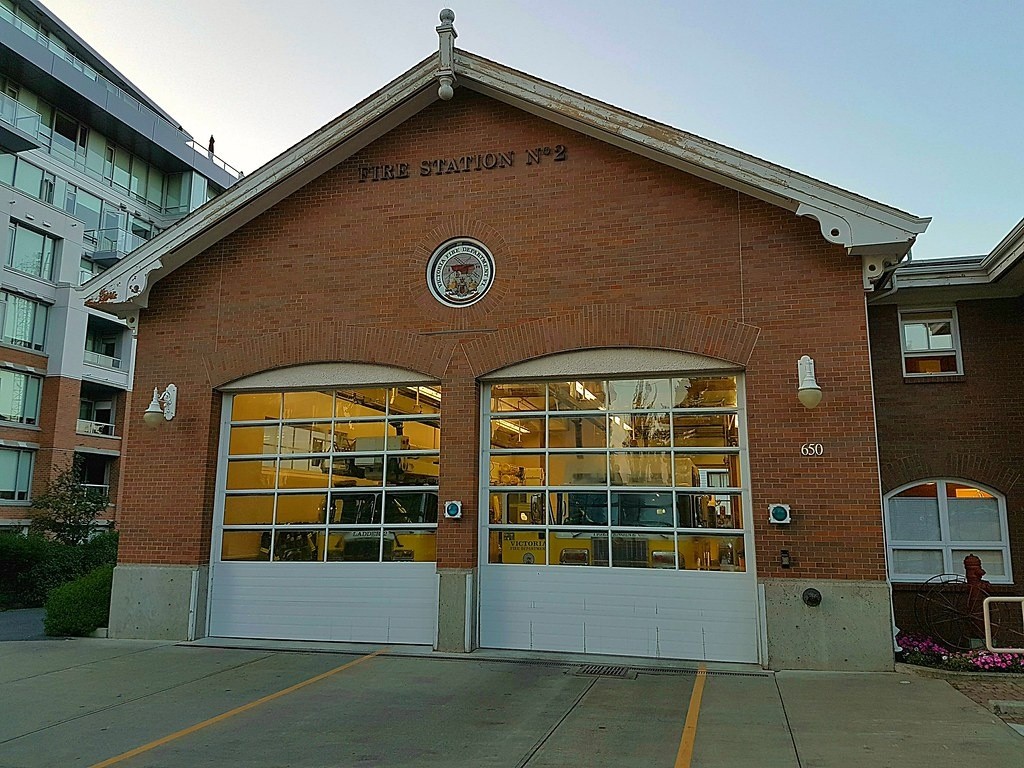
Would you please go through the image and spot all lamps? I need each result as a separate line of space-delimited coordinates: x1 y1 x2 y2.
798 356 822 409
143 383 177 429
408 385 531 434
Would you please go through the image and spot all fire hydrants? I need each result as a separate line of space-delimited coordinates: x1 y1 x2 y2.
964 553 991 638
